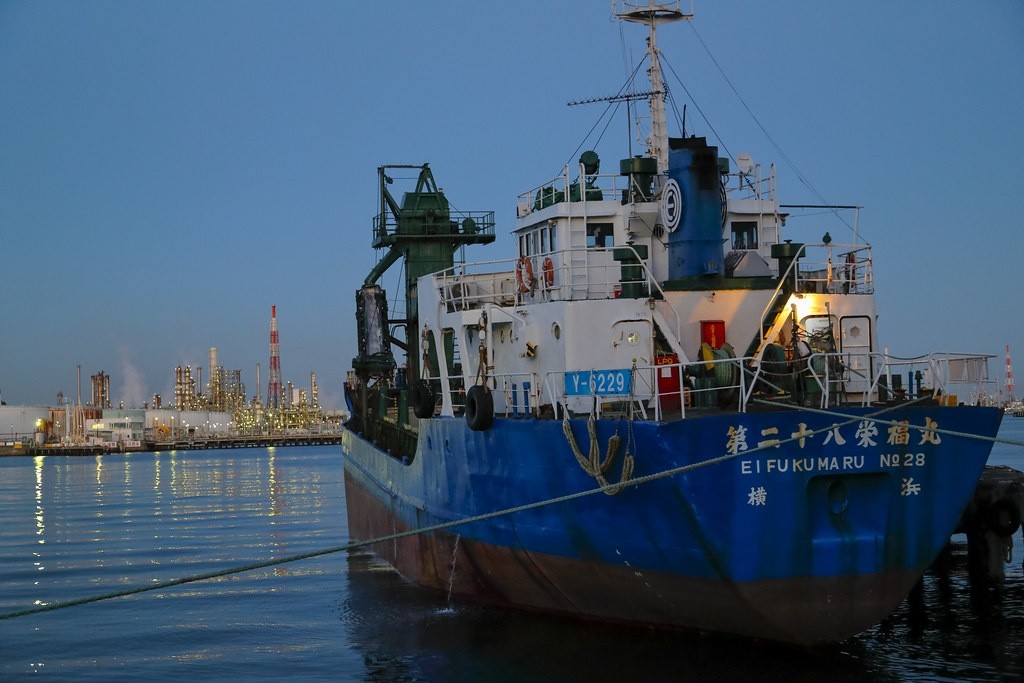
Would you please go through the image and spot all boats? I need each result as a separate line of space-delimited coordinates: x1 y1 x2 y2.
339 1 1007 651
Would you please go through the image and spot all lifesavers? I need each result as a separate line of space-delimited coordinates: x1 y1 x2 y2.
465 385 494 431
411 381 435 419
516 256 532 293
542 257 554 292
